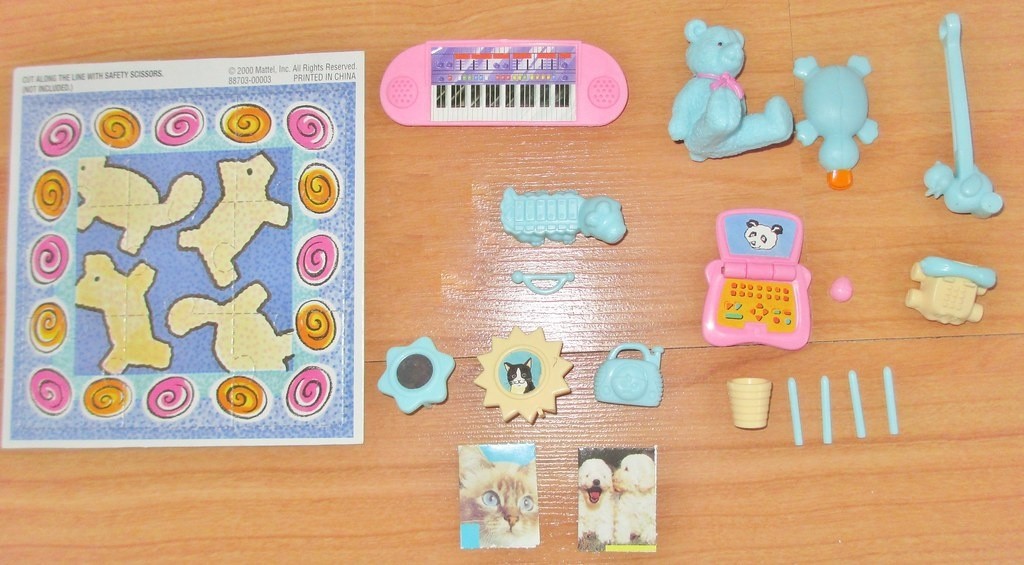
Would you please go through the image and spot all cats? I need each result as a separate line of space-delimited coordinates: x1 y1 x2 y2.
459 442 542 549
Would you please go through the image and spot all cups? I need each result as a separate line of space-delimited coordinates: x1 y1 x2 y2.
727 377 772 429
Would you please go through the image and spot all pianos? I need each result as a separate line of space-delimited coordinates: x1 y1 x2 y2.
380 36 631 129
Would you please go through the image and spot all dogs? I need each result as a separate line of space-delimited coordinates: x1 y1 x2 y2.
579 446 658 552
